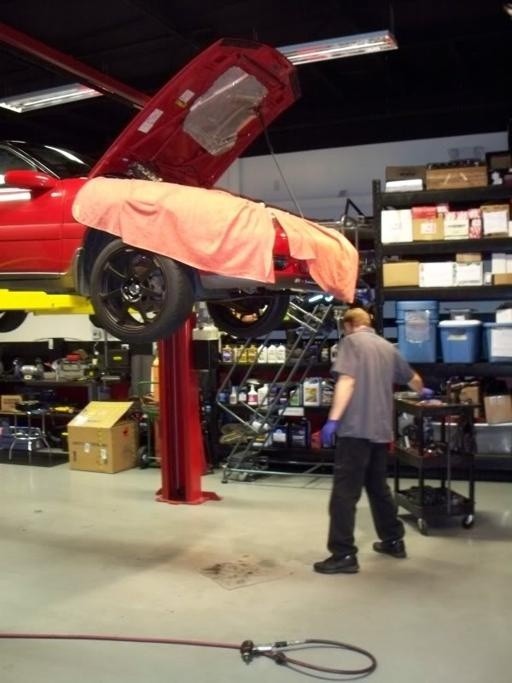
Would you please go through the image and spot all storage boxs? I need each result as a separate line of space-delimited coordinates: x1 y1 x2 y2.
484 394 512 425
66 400 139 474
380 160 512 364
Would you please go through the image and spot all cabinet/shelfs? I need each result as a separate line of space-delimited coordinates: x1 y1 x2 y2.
372 178 512 458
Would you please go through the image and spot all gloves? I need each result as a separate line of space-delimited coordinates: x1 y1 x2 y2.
321 420 340 445
421 388 433 398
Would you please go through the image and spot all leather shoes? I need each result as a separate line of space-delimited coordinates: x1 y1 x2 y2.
315 553 358 572
373 539 406 556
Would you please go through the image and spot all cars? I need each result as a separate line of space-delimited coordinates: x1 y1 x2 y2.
0 34 363 349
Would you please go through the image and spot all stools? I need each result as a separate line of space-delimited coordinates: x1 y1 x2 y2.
8 401 52 461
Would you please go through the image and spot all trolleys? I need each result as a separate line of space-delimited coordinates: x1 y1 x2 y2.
391 387 484 537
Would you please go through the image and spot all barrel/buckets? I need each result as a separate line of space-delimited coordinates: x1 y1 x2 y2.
270 416 289 448
303 378 321 407
258 383 270 405
289 417 311 448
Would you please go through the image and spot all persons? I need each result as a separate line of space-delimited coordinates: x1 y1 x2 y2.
313 306 435 575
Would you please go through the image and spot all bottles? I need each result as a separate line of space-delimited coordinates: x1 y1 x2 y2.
229 385 240 404
221 342 286 363
35 358 44 381
320 340 338 362
